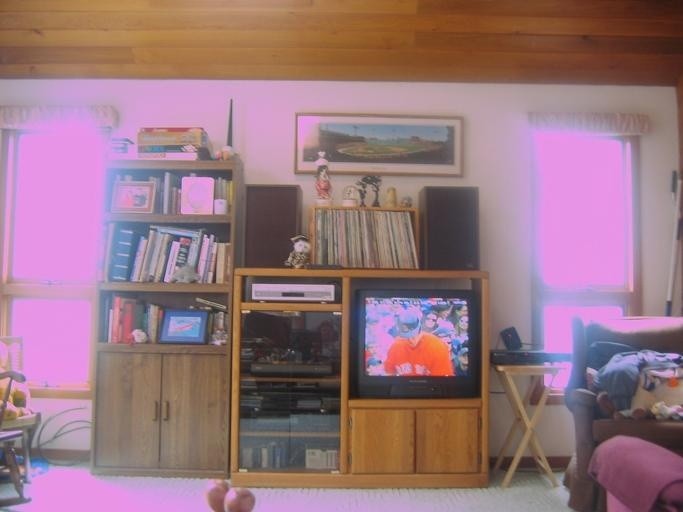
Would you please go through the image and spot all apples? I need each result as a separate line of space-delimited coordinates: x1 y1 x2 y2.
223 487 255 512
209 481 230 512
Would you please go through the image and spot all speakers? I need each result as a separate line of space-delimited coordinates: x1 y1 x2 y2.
418 185 480 271
245 184 302 268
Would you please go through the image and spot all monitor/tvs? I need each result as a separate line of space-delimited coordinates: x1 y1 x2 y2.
355 290 481 399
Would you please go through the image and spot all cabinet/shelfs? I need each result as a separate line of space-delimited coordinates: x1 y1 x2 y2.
229 266 492 488
89 157 244 479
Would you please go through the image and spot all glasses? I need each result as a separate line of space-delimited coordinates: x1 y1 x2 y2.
461 318 468 323
426 318 436 321
445 342 452 345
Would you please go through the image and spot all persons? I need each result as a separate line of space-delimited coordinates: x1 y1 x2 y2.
365 305 469 376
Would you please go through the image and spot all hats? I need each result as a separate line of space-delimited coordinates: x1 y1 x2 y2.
367 358 381 368
432 327 454 337
398 314 420 337
458 348 469 356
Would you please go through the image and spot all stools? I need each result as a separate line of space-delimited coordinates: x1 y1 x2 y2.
490 364 564 487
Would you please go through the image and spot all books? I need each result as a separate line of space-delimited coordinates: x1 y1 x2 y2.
315 208 419 269
102 171 233 344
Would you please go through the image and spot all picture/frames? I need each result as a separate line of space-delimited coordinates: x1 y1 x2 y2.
294 111 464 177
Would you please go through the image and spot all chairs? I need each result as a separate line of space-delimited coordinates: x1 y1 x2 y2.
565 309 683 512
